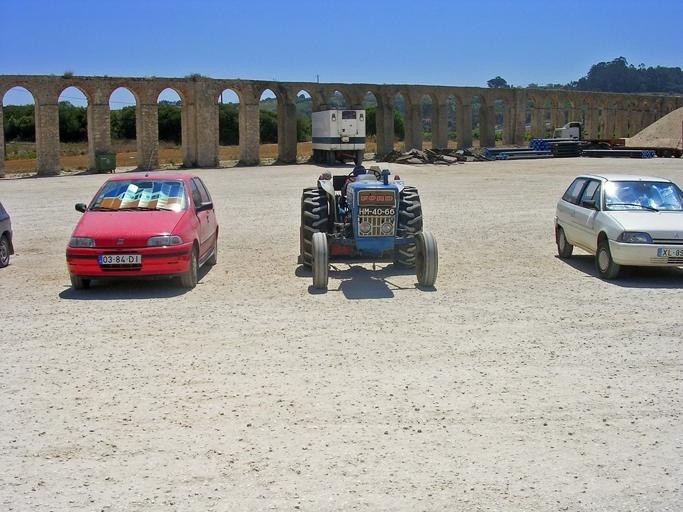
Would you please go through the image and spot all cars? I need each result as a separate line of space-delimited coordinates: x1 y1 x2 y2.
301 166 438 289
0 202 14 268
66 171 219 290
553 174 683 279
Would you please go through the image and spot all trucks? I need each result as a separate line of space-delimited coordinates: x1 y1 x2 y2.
311 110 366 166
553 122 624 148
